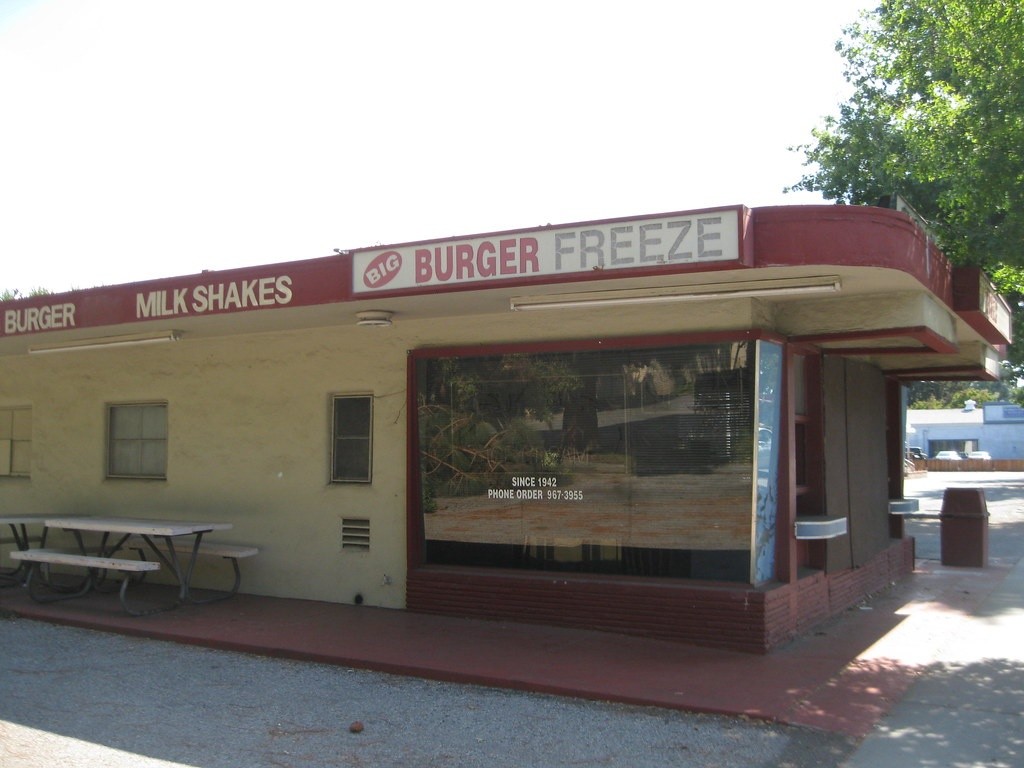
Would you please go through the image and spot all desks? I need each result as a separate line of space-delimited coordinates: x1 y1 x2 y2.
1 512 234 618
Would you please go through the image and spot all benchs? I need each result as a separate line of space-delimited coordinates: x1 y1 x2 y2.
110 536 259 604
9 550 162 615
